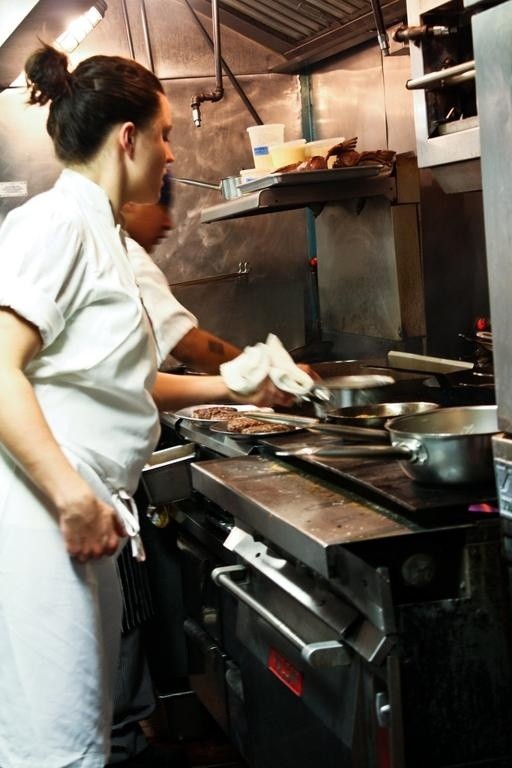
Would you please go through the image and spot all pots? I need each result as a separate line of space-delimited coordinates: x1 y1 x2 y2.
245 399 437 439
285 406 503 486
172 175 243 201
297 365 494 419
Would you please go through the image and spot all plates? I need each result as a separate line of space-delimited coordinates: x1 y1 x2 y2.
210 419 310 438
174 402 261 425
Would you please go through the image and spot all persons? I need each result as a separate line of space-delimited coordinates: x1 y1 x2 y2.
106 168 320 768
0 47 319 768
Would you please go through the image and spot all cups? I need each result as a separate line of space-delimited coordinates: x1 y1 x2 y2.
245 122 283 168
269 137 307 166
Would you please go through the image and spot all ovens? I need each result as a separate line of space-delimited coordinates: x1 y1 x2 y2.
395 1 480 171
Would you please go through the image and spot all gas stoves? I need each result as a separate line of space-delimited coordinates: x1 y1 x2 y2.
174 348 499 564
